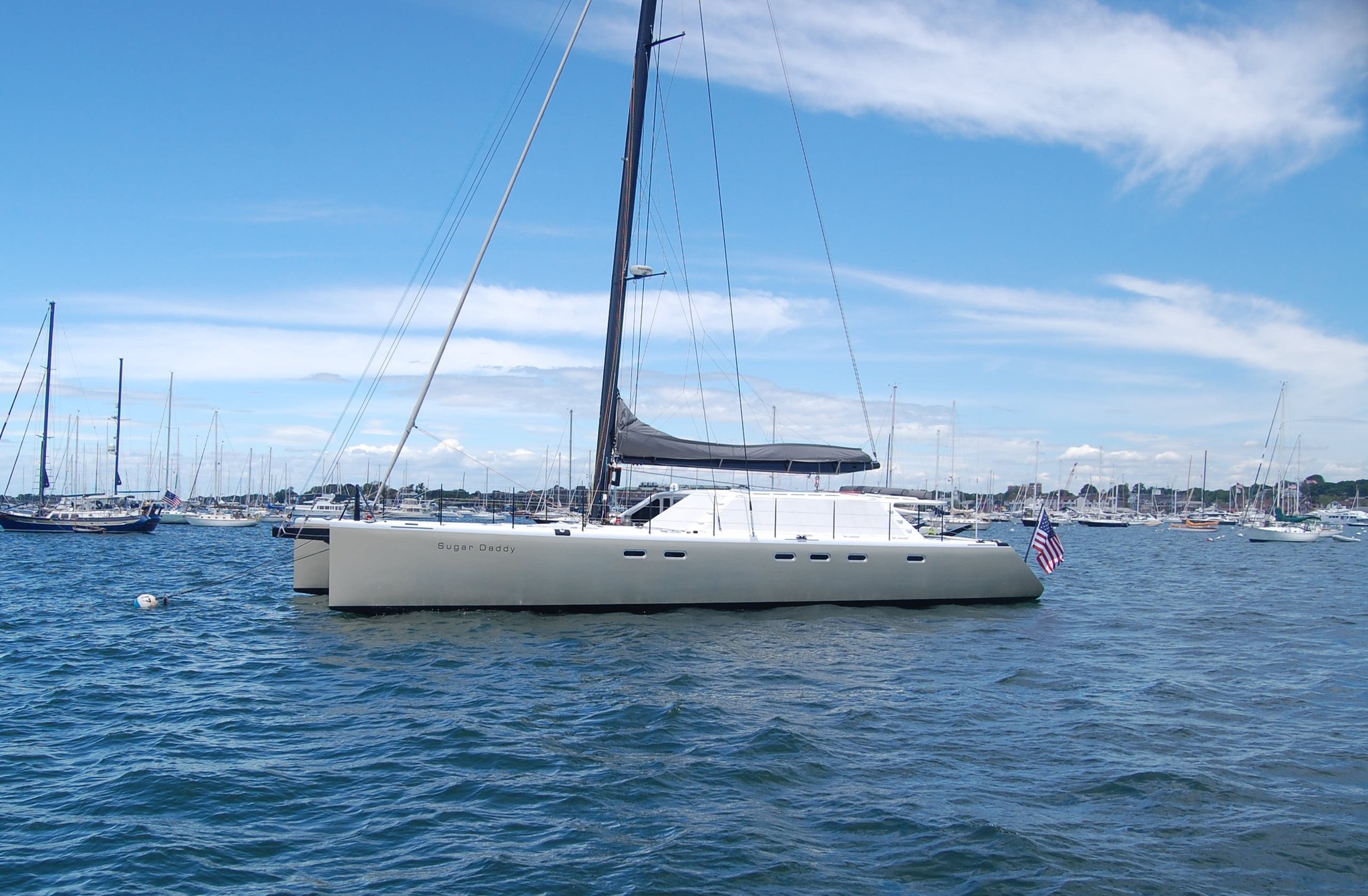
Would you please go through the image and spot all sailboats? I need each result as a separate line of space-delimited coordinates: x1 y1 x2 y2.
266 0 1062 618
0 302 1368 544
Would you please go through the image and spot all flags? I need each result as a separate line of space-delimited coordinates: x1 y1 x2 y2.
162 490 181 508
1030 509 1064 574
